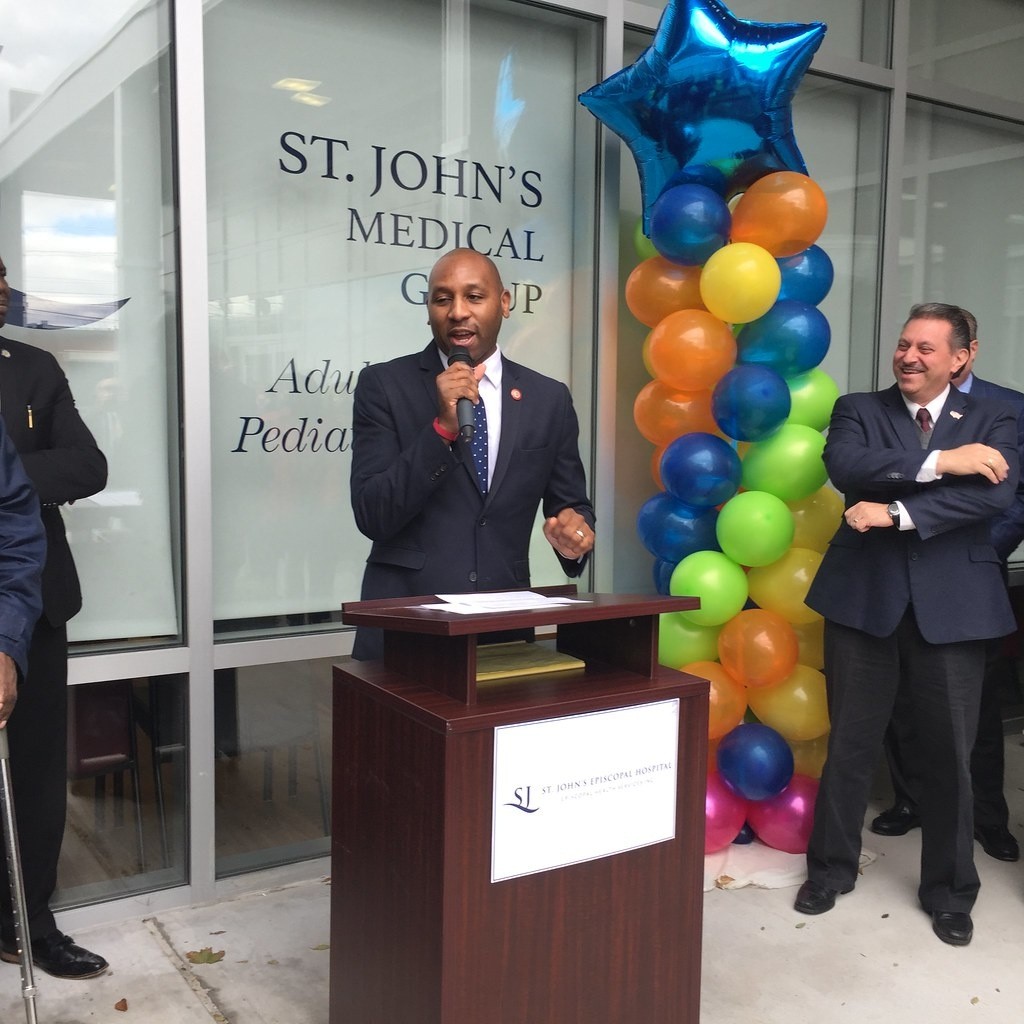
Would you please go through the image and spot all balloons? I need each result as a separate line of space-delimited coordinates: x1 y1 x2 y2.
577 0 845 854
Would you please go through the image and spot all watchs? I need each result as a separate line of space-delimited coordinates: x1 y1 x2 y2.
886 503 900 527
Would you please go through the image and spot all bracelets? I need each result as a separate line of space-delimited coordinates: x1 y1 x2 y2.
434 417 458 440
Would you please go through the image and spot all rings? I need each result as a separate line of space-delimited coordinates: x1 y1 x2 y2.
854 519 858 523
988 458 994 466
576 530 585 538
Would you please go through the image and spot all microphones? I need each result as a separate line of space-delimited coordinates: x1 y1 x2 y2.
447 345 475 443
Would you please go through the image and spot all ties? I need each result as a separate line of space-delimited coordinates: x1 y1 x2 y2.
916 408 931 432
459 392 488 495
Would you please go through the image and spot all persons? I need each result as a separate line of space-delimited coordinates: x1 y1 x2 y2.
0 414 48 731
795 303 1024 946
350 249 596 663
872 309 1024 860
0 257 108 980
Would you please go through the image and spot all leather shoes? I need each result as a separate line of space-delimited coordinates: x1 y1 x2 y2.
931 911 974 945
973 820 1020 861
793 880 855 915
871 803 922 836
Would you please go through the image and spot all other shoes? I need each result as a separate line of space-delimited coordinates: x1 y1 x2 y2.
0 927 109 980
